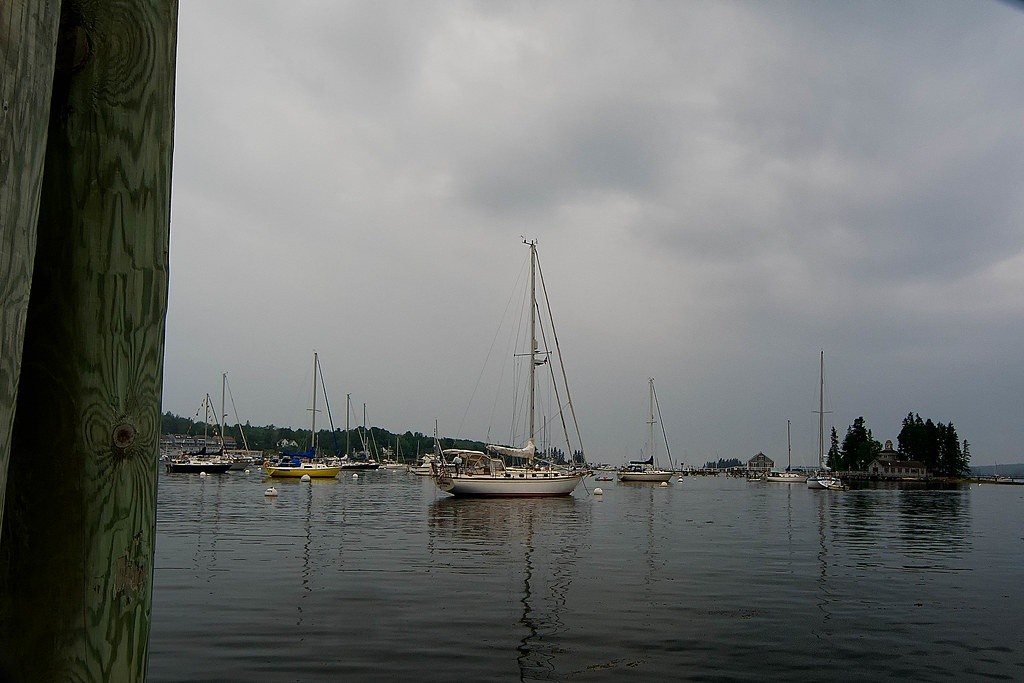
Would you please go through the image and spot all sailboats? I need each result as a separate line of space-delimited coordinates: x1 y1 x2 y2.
163 233 612 498
766 417 809 482
806 349 842 490
616 375 678 482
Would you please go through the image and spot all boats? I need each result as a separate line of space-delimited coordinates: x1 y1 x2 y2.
827 483 851 492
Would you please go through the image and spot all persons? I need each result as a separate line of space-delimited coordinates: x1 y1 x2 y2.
451 454 462 476
